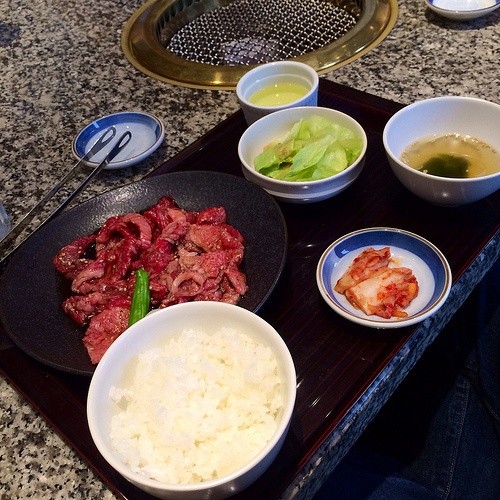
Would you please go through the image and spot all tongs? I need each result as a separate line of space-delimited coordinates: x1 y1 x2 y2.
0 125 127 270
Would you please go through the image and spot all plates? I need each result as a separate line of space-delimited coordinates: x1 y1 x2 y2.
425 0 500 22
72 113 165 168
316 228 452 328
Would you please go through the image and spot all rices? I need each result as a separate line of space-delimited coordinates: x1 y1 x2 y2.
105 330 286 485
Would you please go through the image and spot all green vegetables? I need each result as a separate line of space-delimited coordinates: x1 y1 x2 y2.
255 117 361 182
129 269 150 329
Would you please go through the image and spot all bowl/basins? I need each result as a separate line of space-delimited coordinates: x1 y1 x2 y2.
87 300 297 500
238 106 368 202
383 94 500 207
0 169 289 374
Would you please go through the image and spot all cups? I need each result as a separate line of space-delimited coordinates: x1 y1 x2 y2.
237 59 319 131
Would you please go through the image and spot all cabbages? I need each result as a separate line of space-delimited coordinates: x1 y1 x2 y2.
334 248 418 320
